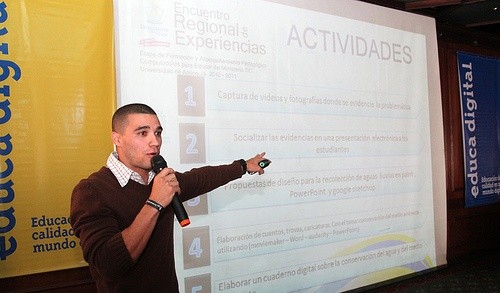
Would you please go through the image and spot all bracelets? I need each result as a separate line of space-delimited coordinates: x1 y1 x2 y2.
240 158 248 175
145 199 164 212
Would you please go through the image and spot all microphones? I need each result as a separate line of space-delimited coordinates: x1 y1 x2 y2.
151 155 190 227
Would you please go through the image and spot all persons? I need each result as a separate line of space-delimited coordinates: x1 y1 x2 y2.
68 103 267 293
